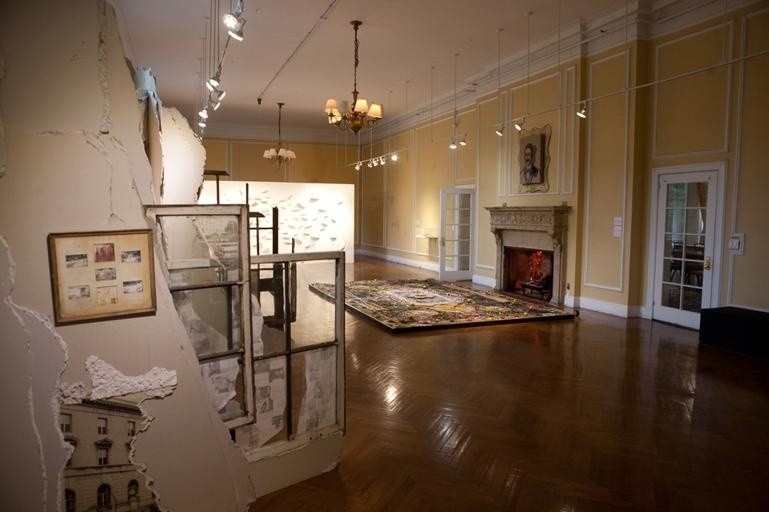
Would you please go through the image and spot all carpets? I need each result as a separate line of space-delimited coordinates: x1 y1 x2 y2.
309 278 576 334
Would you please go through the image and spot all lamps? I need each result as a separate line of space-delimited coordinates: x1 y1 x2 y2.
324 20 383 135
496 124 505 136
577 101 588 119
262 103 296 161
195 0 245 139
514 117 526 132
449 141 457 149
354 152 398 172
459 136 466 146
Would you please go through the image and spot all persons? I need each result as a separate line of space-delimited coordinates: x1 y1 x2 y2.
519 143 541 184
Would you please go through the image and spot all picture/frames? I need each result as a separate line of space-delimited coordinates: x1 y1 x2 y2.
519 133 545 185
46 228 157 327
